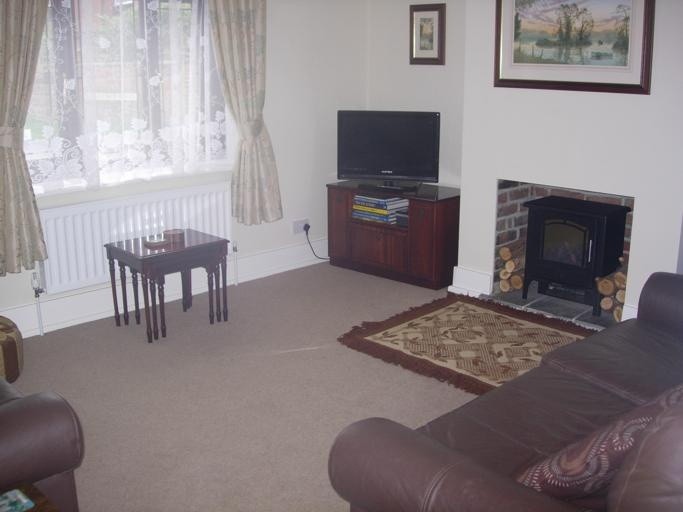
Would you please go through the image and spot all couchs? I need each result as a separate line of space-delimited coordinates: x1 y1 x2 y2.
328 271 682 512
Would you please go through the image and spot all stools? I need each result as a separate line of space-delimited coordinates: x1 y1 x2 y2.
0 314 24 383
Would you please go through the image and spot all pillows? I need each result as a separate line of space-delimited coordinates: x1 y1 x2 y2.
510 383 682 498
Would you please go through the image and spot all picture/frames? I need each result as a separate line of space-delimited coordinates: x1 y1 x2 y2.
408 3 447 67
492 0 655 95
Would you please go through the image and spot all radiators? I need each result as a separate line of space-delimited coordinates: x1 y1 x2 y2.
33 180 237 295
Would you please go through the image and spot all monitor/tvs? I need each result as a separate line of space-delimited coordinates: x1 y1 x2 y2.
336 109 440 190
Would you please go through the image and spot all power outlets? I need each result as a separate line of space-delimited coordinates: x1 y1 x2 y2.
292 219 309 234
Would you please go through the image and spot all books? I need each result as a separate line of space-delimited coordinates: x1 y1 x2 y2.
352 193 410 226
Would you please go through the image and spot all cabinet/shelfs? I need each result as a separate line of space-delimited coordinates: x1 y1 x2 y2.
326 178 461 291
521 196 631 317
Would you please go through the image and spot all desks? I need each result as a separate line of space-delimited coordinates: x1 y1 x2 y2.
104 229 231 343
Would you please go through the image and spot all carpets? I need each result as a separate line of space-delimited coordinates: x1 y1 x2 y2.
337 292 601 395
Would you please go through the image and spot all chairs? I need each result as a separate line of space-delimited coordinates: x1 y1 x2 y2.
0 375 86 512
